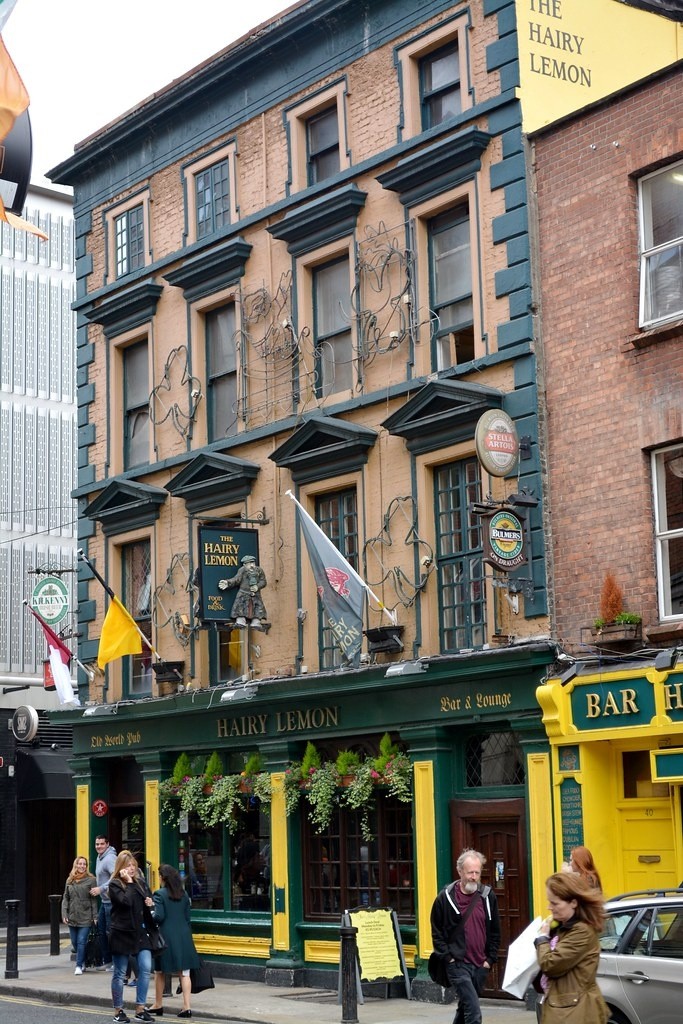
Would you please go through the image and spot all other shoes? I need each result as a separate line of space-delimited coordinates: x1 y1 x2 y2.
128 979 138 987
176 987 182 994
97 962 114 972
123 980 128 985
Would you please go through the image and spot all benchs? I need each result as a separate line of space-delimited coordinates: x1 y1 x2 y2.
640 940 683 959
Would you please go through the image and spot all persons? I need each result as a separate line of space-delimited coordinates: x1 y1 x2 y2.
430 850 500 1024
108 850 156 1024
123 954 138 987
217 555 267 629
533 872 612 1024
570 846 601 890
87 834 118 972
61 857 99 974
187 834 414 918
145 864 198 1018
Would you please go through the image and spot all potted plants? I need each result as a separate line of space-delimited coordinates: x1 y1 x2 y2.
590 572 642 641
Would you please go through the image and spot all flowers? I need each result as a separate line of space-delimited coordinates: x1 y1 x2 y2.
157 732 413 842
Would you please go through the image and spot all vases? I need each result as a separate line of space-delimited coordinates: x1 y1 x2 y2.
166 770 395 796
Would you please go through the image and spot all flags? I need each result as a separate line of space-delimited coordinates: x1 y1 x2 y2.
295 502 365 670
80 553 142 671
26 604 80 707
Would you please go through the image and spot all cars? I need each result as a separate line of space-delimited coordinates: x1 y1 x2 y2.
534 888 683 1024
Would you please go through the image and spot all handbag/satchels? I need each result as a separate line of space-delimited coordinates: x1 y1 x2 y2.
149 924 167 955
190 961 215 993
85 927 102 967
502 915 552 999
428 952 452 989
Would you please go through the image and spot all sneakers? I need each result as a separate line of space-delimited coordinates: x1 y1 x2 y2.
113 1010 130 1023
75 963 87 974
134 1009 155 1023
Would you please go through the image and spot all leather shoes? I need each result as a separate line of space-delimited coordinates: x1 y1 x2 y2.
144 1006 163 1015
177 1009 192 1018
